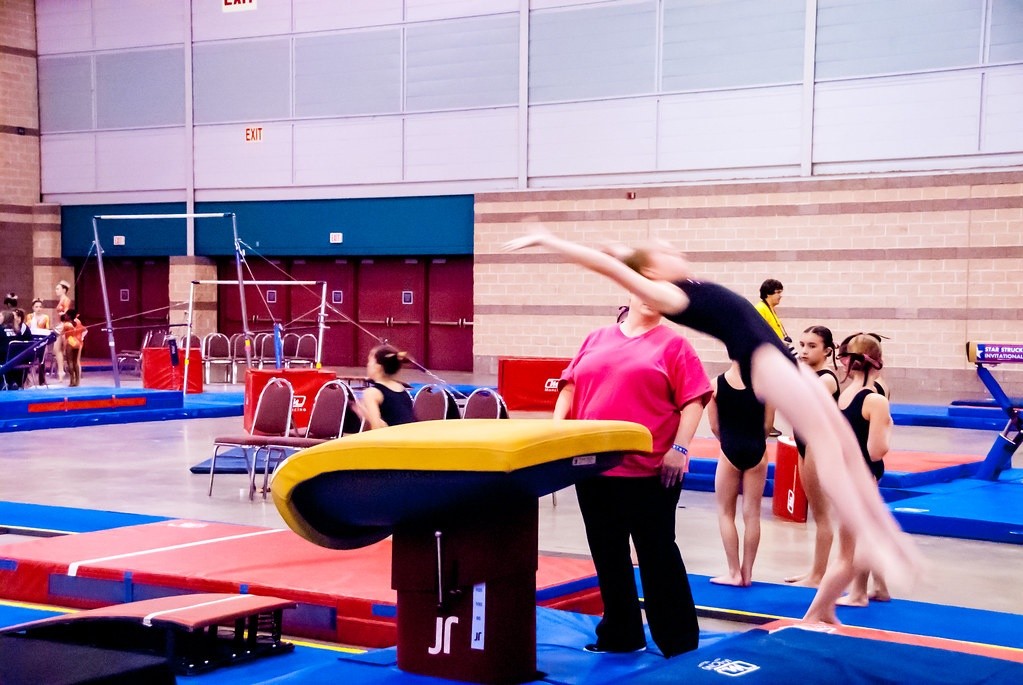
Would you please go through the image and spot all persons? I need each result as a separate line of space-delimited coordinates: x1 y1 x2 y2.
0 280 88 387
553 285 714 660
705 279 894 626
351 346 416 432
499 217 940 600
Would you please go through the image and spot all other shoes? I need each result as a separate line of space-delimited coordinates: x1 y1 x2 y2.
769 427 782 437
582 644 646 653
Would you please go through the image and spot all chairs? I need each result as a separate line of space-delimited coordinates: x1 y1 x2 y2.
497 393 511 419
208 377 294 497
336 379 366 433
463 388 501 421
443 389 462 419
412 383 447 422
249 379 349 502
116 329 317 385
1 340 40 391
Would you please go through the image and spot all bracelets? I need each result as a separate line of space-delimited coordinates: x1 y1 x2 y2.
672 444 688 455
